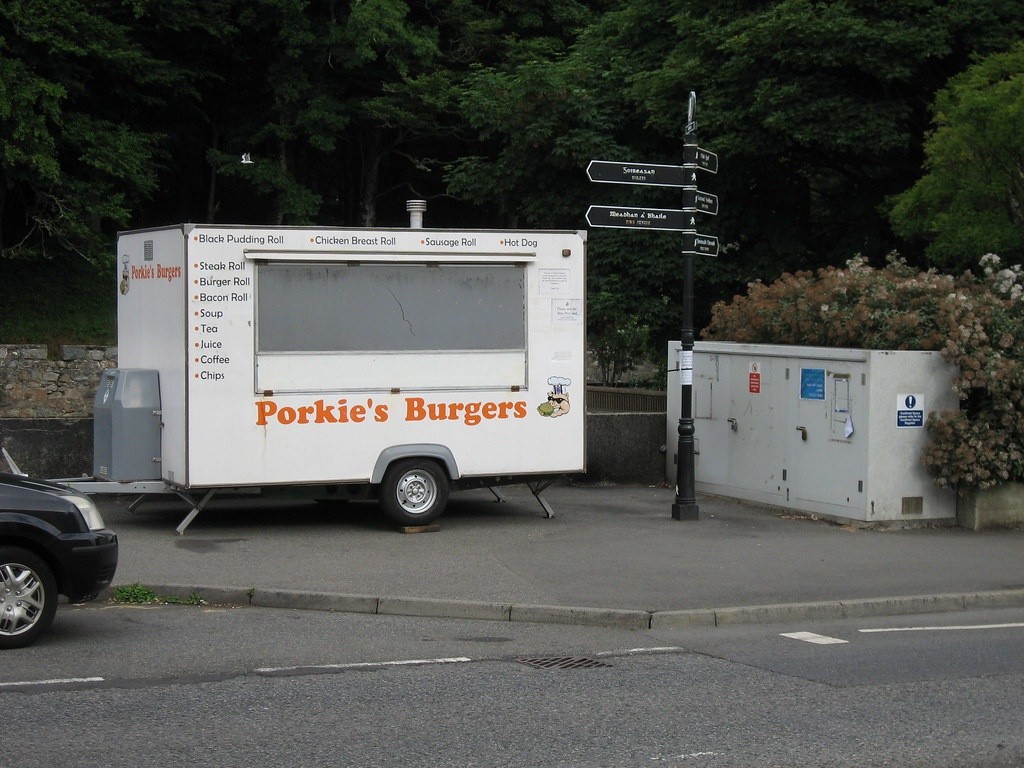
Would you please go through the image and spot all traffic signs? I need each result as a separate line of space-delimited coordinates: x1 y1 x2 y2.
585 160 698 187
683 234 719 256
683 187 720 215
584 205 699 232
685 146 719 174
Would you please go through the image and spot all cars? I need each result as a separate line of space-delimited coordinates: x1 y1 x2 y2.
1 469 119 650
